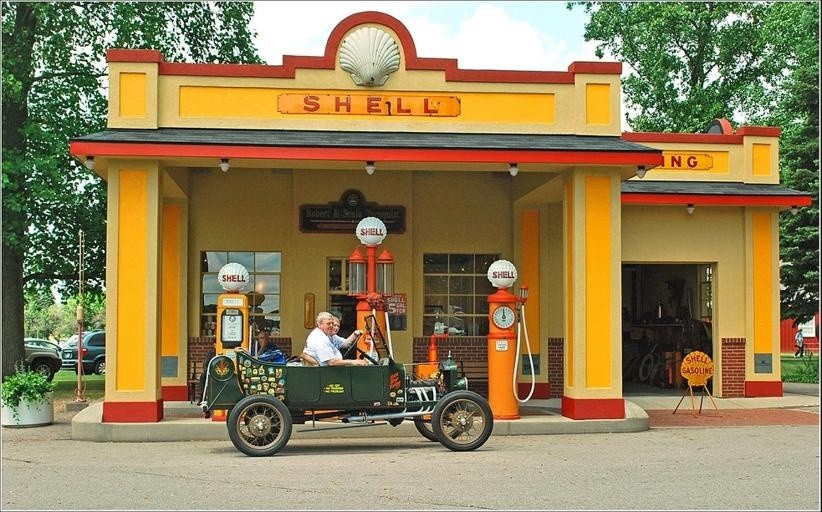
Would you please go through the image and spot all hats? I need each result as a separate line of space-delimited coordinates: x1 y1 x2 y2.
797 328 802 332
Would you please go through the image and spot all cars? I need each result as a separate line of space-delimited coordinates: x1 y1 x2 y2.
23 338 62 382
197 313 493 455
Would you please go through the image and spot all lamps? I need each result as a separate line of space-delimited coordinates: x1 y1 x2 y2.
508 162 519 177
84 155 96 170
686 203 695 215
219 158 230 173
364 161 376 175
635 166 647 179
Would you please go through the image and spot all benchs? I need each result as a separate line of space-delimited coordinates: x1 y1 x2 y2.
457 361 489 384
188 361 204 403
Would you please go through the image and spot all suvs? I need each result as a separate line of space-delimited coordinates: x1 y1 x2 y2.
60 329 105 375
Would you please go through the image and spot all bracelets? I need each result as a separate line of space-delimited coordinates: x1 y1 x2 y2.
353 332 358 337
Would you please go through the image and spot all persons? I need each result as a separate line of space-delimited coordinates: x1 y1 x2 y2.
302 312 366 366
793 327 805 358
196 337 216 405
328 315 364 351
252 332 282 356
